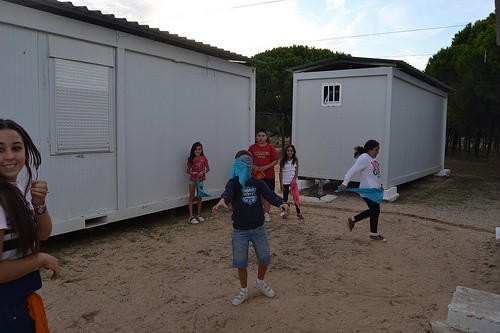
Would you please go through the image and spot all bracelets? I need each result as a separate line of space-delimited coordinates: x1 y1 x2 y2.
31 199 46 215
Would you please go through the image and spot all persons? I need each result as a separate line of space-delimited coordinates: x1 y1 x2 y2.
279 145 305 220
247 129 279 222
0 119 60 333
212 150 291 306
186 141 210 225
340 140 387 243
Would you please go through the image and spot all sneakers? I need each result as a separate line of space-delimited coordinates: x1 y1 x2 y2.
196 215 204 221
369 234 387 242
188 217 199 224
297 214 304 220
282 214 288 220
254 280 274 297
346 218 354 232
232 290 248 306
264 214 271 222
